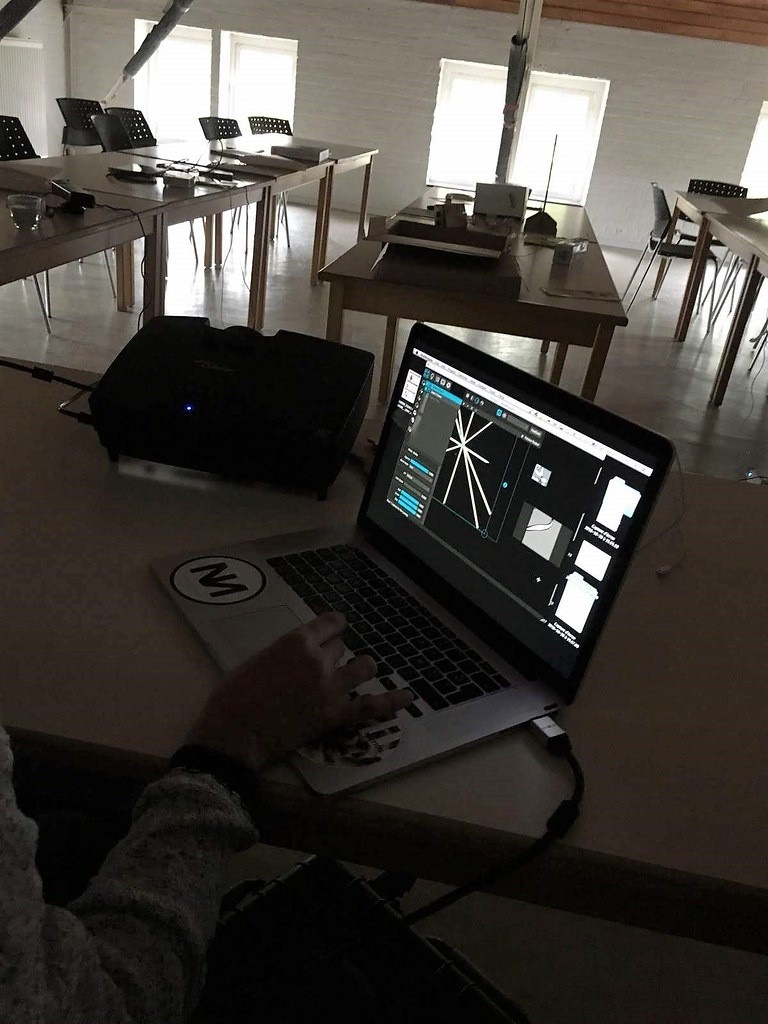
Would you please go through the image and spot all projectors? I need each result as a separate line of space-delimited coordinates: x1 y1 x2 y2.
87 315 378 502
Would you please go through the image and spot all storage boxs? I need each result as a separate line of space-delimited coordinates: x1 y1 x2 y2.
553 237 589 265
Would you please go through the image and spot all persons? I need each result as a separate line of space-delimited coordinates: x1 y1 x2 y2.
0 608 415 1024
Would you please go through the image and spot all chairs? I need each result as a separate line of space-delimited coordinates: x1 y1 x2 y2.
622 179 768 371
0 98 294 334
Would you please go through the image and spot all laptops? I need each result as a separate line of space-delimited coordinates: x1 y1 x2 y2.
150 324 678 801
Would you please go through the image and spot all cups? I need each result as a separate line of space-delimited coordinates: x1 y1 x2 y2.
7 194 44 232
552 241 574 267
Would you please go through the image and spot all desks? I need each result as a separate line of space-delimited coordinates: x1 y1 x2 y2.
2 357 768 955
318 185 628 402
0 133 379 330
651 190 768 406
